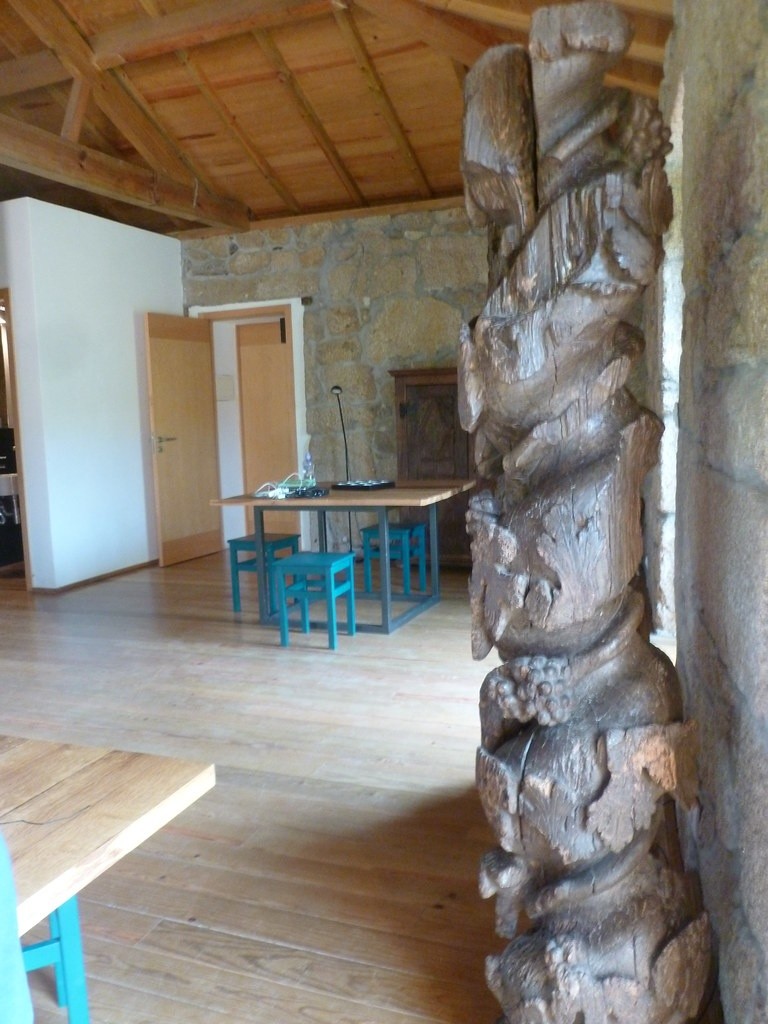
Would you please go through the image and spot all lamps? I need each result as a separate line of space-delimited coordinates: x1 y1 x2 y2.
330 386 352 553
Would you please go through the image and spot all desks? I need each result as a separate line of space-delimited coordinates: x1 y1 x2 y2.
210 478 477 635
0 734 217 940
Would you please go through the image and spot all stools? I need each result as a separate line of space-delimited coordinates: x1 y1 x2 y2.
226 530 303 615
271 550 356 652
22 895 92 1023
360 522 427 596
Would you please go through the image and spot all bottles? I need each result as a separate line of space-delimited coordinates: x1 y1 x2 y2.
303 450 316 488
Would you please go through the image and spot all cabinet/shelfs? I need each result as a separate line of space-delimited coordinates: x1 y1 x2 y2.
390 368 476 569
